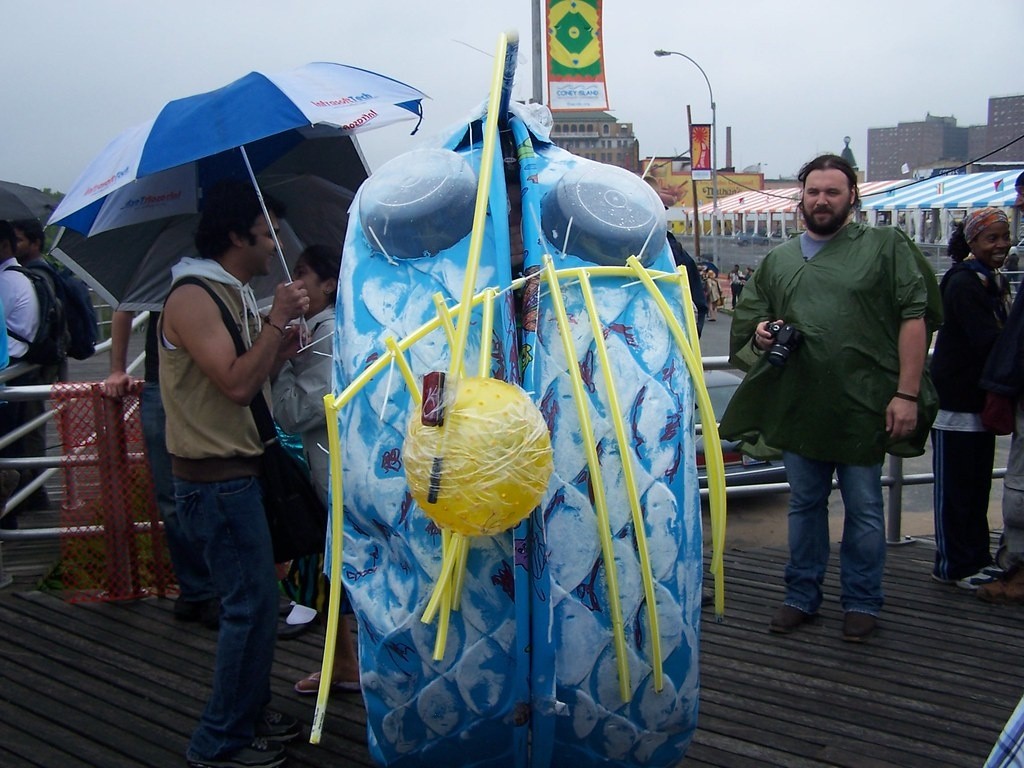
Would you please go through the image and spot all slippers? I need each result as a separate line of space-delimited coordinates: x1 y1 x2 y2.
295 669 359 693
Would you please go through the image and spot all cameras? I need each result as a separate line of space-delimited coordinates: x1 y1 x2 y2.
765 323 805 366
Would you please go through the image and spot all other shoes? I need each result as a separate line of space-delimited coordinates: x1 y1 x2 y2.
977 579 1006 603
0 572 13 589
1005 571 1024 600
276 617 315 642
15 498 50 512
173 595 223 631
1 517 18 530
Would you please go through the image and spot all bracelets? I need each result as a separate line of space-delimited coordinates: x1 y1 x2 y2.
894 392 918 402
264 315 283 333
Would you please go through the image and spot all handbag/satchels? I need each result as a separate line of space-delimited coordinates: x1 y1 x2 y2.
716 297 725 305
253 441 327 563
979 391 1014 436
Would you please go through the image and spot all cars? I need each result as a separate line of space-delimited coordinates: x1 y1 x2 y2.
694 355 787 505
734 225 804 247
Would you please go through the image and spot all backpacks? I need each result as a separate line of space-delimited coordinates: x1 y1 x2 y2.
6 266 71 383
26 261 100 360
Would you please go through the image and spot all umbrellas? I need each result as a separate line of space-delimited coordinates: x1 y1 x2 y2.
47 134 372 312
42 60 433 282
0 180 56 222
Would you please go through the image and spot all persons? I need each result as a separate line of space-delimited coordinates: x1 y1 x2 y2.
272 245 360 693
727 264 755 310
977 171 1024 604
926 205 1014 590
719 155 945 640
0 217 63 530
155 185 310 768
104 310 222 628
697 265 720 322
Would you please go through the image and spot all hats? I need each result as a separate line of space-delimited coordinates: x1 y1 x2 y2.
301 245 342 277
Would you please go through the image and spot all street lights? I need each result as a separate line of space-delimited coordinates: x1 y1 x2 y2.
653 49 718 265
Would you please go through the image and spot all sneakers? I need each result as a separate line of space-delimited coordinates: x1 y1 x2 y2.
186 743 286 768
932 569 997 591
979 565 1005 579
254 707 302 741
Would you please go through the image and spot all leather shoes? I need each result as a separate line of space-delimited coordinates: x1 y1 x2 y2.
768 605 813 632
842 611 876 641
